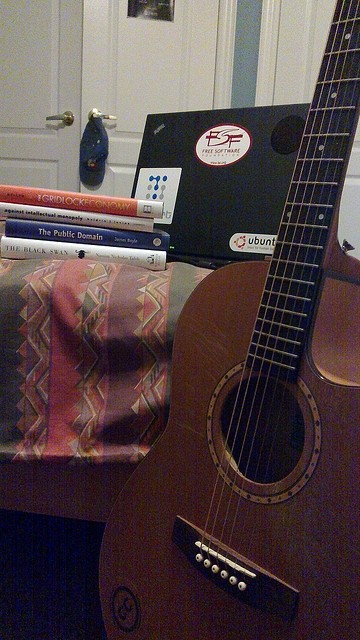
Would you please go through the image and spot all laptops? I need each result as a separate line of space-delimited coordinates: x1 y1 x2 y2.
131 103 311 270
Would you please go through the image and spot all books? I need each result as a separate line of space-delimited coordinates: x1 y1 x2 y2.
1 236 167 272
0 202 154 233
1 182 164 218
4 219 171 251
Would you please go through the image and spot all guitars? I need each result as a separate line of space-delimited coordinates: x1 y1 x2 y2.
97 0 360 639
